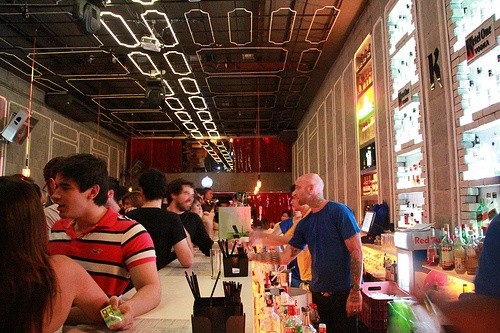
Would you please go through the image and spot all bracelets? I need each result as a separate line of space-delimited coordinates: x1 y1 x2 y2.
266 234 269 241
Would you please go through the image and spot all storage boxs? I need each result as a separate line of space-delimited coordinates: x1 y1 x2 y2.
218 207 252 242
358 280 413 333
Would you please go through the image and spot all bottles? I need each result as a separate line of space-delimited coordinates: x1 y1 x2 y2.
210 249 222 279
426 199 499 275
383 253 398 283
403 160 421 186
250 244 326 333
403 201 424 228
361 173 379 195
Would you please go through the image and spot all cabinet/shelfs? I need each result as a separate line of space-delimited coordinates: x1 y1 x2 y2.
353 0 500 247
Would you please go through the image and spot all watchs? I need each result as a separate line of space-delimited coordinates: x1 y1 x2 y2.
349 284 363 293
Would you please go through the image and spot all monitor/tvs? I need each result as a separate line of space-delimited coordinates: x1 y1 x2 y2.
362 210 375 233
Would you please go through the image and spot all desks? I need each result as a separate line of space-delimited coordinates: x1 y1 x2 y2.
422 263 476 286
68 242 255 333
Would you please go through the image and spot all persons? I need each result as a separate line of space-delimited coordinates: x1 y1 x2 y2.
45 154 161 330
0 174 109 333
201 203 219 229
231 173 363 333
214 197 230 224
42 157 63 230
165 178 240 257
15 174 54 209
190 195 213 240
195 187 214 205
121 168 193 295
249 185 312 304
269 200 301 288
410 285 500 333
106 177 144 215
477 215 500 298
271 210 294 236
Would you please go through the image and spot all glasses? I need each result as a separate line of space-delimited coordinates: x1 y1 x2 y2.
42 177 55 193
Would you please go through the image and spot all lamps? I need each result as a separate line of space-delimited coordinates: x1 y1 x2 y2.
0 109 40 143
144 79 165 108
